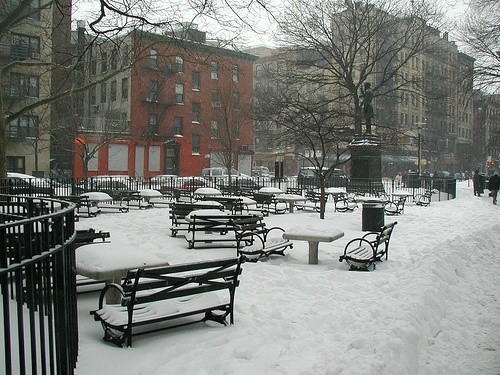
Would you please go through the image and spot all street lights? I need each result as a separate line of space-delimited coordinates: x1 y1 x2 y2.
415 121 427 173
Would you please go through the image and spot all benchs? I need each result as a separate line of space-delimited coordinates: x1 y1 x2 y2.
202 196 246 215
334 191 365 211
412 190 437 207
383 197 406 216
76 188 294 348
339 221 398 272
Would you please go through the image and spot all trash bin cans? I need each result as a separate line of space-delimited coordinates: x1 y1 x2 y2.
405 172 419 187
361 203 385 232
476 175 486 194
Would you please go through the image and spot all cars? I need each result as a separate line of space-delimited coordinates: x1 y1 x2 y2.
149 174 220 199
251 165 269 176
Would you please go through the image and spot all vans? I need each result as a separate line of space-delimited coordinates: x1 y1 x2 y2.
297 166 349 188
6 172 55 194
77 176 135 199
201 167 252 186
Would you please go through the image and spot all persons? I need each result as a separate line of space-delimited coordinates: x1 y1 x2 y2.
473 170 482 197
359 82 374 134
464 171 471 180
396 169 455 189
488 174 500 205
458 171 464 182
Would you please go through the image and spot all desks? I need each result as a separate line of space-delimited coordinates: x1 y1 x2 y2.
392 192 414 197
326 187 347 196
185 210 264 225
283 219 344 265
81 190 113 213
259 186 286 195
220 194 256 207
75 241 170 305
184 211 263 225
192 199 223 211
278 195 307 212
194 186 222 196
132 187 162 208
